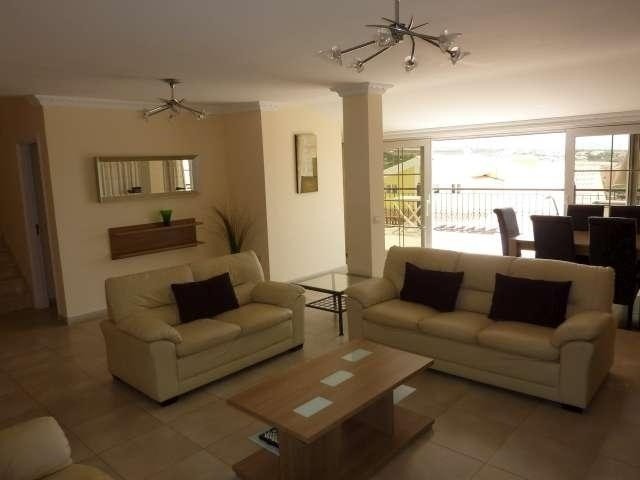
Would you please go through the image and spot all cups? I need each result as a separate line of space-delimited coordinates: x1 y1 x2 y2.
160 210 173 226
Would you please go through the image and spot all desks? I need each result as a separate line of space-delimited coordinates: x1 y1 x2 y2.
392 196 422 233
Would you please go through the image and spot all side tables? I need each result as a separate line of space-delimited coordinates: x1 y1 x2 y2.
296 272 371 336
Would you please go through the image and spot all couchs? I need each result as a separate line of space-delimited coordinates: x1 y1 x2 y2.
345 245 618 414
0 416 114 480
99 250 305 407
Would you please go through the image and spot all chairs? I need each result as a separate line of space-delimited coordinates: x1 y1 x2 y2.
493 204 640 326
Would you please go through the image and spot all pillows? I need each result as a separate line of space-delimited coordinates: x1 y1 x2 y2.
170 272 240 324
399 262 464 312
487 273 572 329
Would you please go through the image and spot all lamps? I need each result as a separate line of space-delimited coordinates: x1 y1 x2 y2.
141 79 207 123
313 0 471 75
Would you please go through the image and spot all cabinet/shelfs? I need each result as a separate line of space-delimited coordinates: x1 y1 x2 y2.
109 218 206 260
